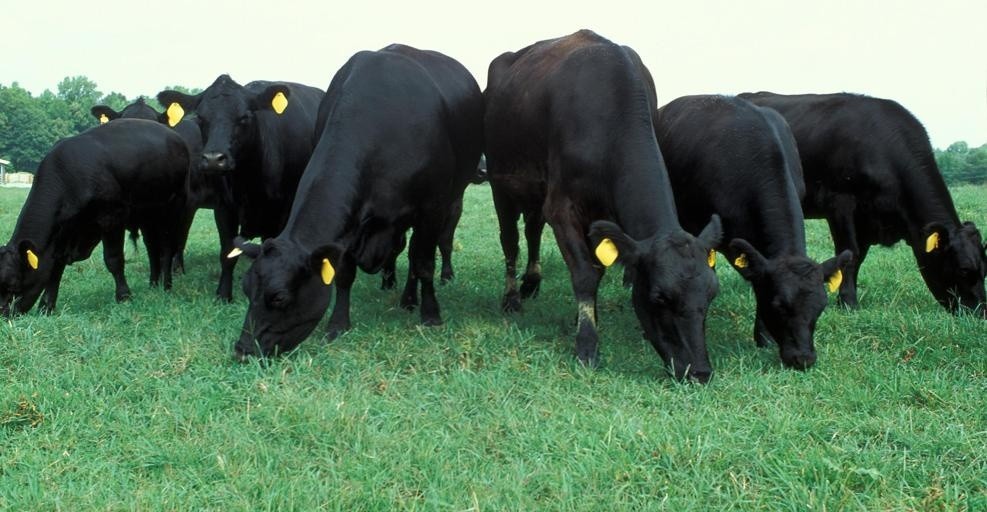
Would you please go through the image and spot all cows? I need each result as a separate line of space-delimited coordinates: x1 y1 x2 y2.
88 92 210 290
734 88 987 320
236 44 482 369
0 113 190 320
154 72 327 304
479 27 724 383
658 92 854 371
